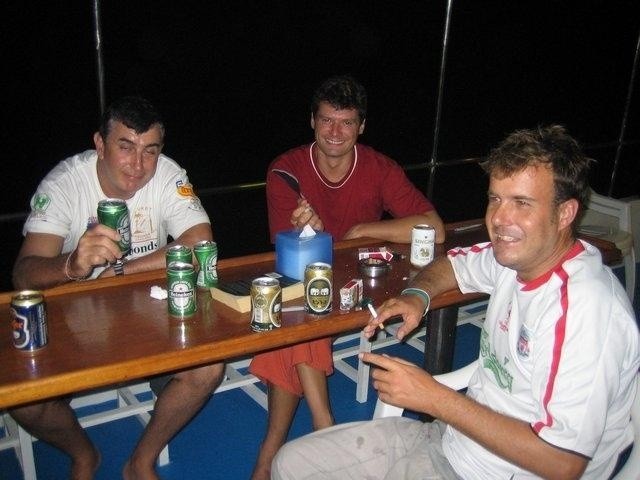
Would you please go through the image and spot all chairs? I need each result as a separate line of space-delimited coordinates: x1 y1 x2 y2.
575 186 636 305
373 358 640 480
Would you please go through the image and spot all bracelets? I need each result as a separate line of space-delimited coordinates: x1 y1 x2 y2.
65 249 78 280
401 288 431 316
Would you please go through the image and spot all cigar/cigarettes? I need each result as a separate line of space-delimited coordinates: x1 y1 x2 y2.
367 304 385 330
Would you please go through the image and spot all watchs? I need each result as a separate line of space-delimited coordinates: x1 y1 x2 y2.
112 258 127 276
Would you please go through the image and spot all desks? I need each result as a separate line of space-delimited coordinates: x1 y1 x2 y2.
0 218 624 423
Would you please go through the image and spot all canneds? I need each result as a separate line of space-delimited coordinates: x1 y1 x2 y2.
250 275 280 334
96 198 132 258
11 291 49 353
166 237 218 319
409 224 436 268
303 262 335 317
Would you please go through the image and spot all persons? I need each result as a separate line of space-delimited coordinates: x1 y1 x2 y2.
251 77 447 479
270 122 638 480
9 91 227 480
